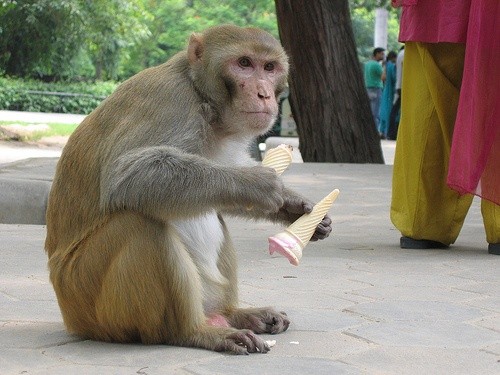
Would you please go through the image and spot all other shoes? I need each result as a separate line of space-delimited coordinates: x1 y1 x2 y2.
488 243 500 255
400 236 449 250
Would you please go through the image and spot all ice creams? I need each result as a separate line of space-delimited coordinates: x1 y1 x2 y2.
246 144 294 211
267 189 339 266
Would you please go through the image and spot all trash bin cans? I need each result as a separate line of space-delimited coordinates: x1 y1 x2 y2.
279 94 298 137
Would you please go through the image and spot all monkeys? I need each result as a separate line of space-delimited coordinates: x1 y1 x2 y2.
40 22 334 357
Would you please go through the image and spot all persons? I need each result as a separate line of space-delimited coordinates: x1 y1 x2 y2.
387 47 404 141
391 0 500 255
376 51 396 138
364 47 385 116
